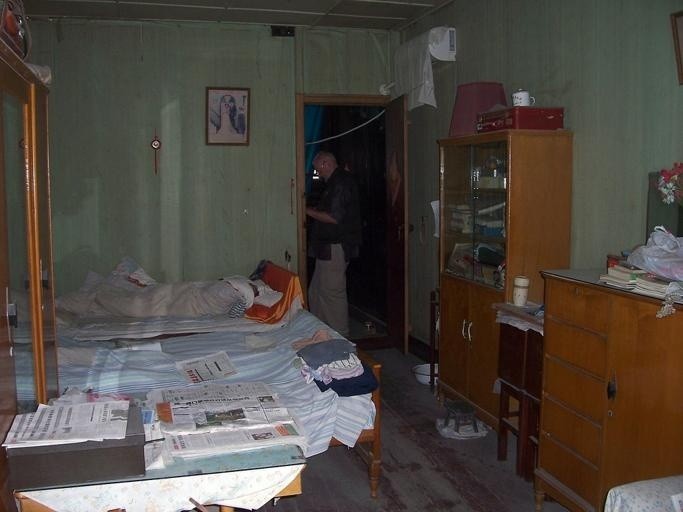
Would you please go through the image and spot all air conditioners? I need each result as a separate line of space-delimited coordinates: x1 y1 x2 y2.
394 27 458 70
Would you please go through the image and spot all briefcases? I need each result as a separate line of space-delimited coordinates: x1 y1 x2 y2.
476 107 565 132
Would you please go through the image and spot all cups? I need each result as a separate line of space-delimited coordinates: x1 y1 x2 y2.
512 275 530 307
511 88 536 106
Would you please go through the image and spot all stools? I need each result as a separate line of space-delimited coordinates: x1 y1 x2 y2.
444 402 479 433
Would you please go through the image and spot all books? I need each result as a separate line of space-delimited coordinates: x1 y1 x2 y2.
598 257 682 304
444 174 506 236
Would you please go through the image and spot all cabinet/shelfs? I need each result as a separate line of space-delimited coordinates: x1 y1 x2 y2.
437 131 574 433
0 38 60 512
532 267 683 512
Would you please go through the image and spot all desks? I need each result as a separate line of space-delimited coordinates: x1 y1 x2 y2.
12 385 311 512
496 313 544 482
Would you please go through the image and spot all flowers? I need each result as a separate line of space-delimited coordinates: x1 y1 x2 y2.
656 161 683 210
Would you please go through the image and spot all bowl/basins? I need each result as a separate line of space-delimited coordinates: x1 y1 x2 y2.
413 363 440 386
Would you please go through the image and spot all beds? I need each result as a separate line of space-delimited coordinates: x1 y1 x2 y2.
56 261 306 342
58 311 384 499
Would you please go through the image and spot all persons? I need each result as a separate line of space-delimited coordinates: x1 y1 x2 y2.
303 147 363 343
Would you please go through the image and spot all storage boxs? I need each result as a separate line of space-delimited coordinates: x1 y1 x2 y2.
7 407 147 491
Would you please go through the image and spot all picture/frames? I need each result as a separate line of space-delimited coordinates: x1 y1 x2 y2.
670 10 683 85
205 86 250 146
